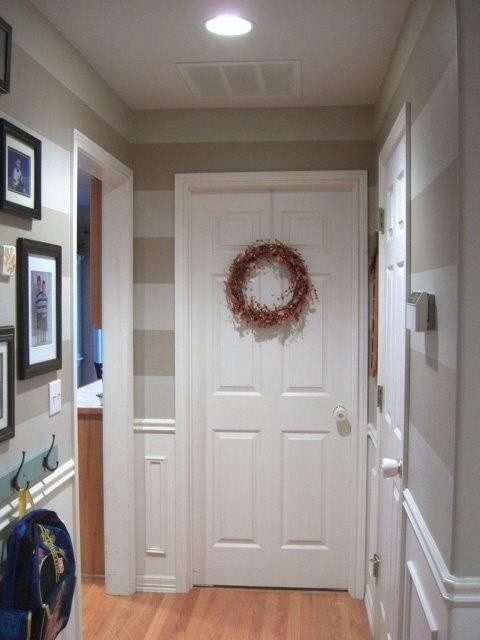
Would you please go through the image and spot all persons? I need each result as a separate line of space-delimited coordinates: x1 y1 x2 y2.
12 154 24 192
35 275 48 344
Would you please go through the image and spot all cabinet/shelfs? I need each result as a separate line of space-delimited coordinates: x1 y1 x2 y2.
78 378 105 589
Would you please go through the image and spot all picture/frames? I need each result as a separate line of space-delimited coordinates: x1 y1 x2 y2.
1 15 61 442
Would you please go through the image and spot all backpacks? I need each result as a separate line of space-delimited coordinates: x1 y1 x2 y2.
0 509 76 640
